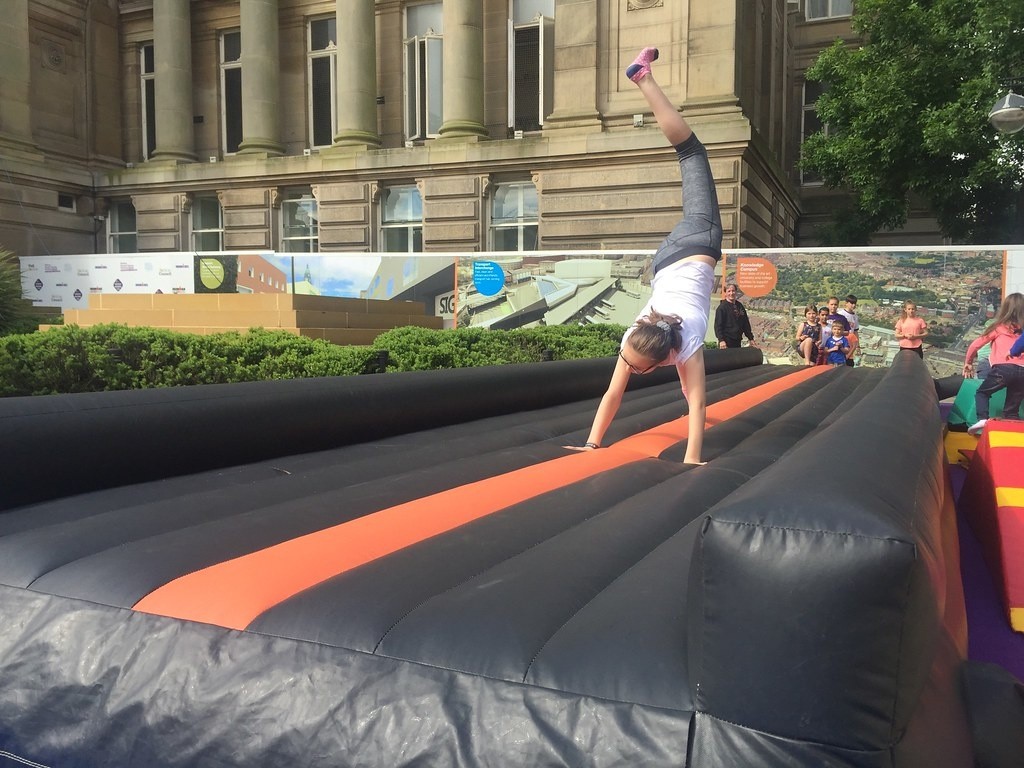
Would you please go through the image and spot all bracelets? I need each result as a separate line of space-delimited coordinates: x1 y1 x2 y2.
903 334 905 338
585 443 599 449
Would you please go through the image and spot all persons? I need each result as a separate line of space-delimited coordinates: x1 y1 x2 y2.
826 297 851 336
837 295 859 340
842 330 858 367
963 293 1024 434
714 284 759 350
560 47 724 465
817 307 835 365
795 305 822 366
894 300 928 360
824 320 850 365
1004 328 1024 362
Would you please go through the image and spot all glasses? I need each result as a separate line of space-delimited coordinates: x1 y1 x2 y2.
618 349 658 376
